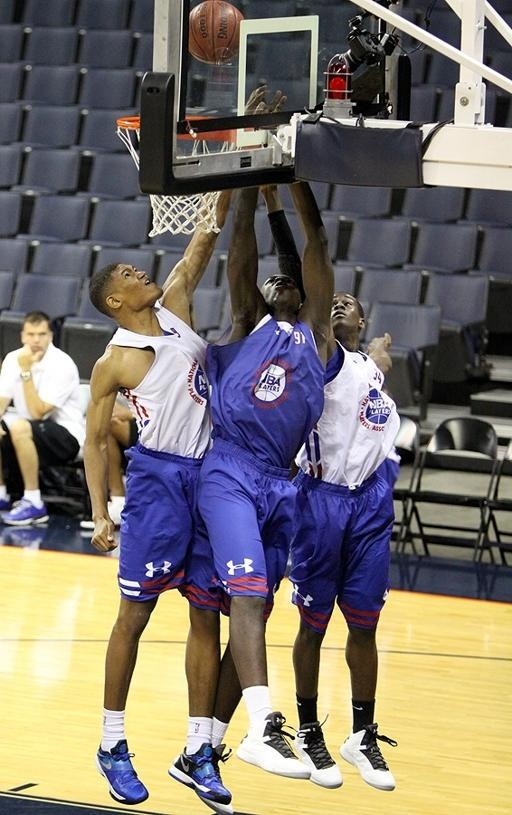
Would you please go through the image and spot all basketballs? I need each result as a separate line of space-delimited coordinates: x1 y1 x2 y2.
189 0 243 61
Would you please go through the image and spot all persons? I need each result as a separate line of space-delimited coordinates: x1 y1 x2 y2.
83 82 271 806
0 311 87 526
196 88 336 815
80 402 140 530
259 184 403 792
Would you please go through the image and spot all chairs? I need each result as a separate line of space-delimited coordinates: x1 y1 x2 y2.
2 0 512 564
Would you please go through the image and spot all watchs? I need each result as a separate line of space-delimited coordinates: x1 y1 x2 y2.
19 371 33 378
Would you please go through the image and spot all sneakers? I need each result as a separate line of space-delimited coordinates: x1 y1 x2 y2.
339 724 395 790
0 494 49 525
168 744 235 815
236 712 311 779
95 740 148 805
80 502 123 529
295 722 343 789
